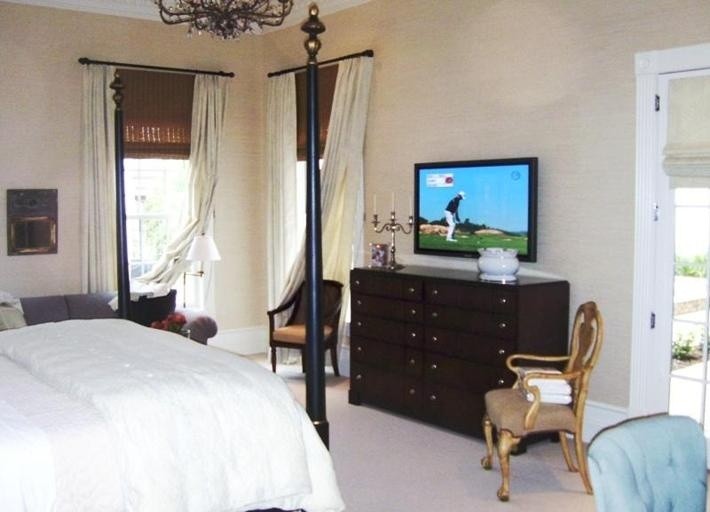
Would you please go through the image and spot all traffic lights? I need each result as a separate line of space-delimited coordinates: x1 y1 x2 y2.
0 304 24 331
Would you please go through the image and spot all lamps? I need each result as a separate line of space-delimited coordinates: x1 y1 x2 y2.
585 411 707 512
267 279 344 373
480 301 604 501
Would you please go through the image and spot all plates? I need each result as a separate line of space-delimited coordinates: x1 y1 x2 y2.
349 265 569 456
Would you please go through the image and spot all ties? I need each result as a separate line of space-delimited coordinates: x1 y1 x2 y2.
414 157 539 264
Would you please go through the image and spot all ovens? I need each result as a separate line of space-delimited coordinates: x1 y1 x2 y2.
0 318 347 512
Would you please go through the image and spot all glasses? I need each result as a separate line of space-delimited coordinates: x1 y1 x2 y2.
150 0 295 40
173 232 222 308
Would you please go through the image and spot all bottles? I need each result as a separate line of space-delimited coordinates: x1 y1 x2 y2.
459 192 464 199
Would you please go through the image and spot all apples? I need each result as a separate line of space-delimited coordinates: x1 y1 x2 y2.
409 194 412 217
391 192 395 213
373 193 377 214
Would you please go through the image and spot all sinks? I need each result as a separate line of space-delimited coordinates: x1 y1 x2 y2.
20 292 217 344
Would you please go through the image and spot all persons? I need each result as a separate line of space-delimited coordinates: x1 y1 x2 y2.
443 191 467 242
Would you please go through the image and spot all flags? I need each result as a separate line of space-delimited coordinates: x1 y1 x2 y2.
477 248 520 282
